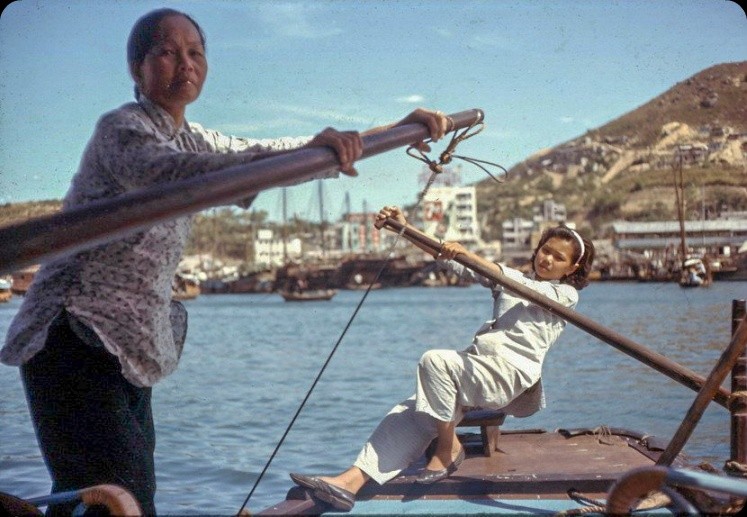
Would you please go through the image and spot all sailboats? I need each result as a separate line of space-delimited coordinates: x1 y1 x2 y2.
668 149 709 289
157 177 468 304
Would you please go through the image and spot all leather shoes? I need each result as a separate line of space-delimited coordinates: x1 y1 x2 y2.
415 447 466 485
287 473 353 513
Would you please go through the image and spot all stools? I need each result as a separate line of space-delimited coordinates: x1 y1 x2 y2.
424 407 507 456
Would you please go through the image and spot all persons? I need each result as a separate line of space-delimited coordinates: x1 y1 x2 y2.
290 205 595 513
0 8 448 517
